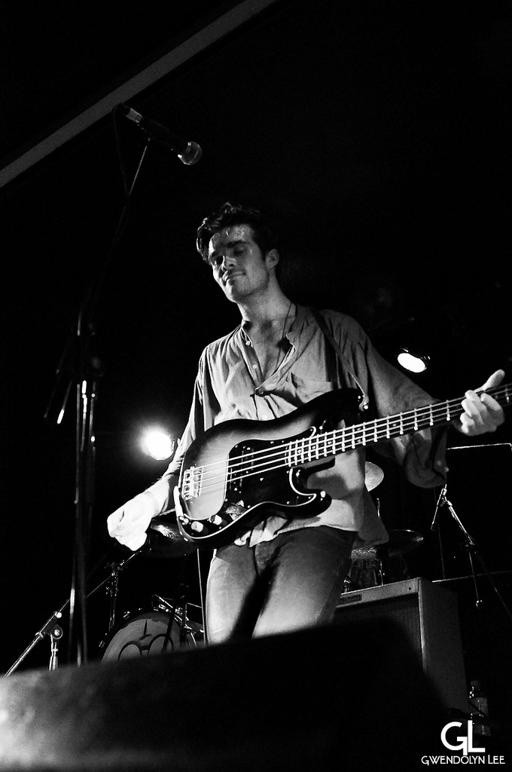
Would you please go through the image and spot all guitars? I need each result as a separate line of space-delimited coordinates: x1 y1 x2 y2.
173 385 511 550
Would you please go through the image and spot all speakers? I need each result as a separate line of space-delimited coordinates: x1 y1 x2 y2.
332 576 471 743
0 615 494 772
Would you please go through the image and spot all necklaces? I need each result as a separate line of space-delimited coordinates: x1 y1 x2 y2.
275 299 293 370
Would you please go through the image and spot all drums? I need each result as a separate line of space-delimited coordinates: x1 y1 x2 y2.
96 610 205 663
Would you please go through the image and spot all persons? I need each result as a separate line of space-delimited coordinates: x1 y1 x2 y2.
104 203 512 649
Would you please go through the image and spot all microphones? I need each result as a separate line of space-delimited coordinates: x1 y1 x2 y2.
429 483 448 533
119 102 203 166
